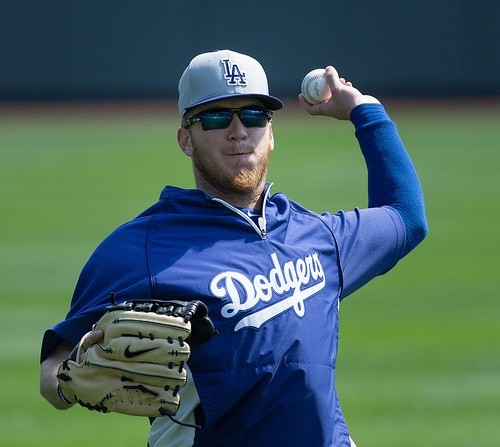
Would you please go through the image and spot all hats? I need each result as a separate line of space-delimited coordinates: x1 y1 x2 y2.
177 49 283 115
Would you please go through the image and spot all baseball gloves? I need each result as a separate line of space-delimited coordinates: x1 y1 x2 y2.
56 293 214 429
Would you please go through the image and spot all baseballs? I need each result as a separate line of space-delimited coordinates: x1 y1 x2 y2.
302 68 336 104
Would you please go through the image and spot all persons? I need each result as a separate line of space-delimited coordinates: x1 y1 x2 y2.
40 48 430 447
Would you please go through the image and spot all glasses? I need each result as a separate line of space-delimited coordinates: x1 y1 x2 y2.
179 105 275 131
